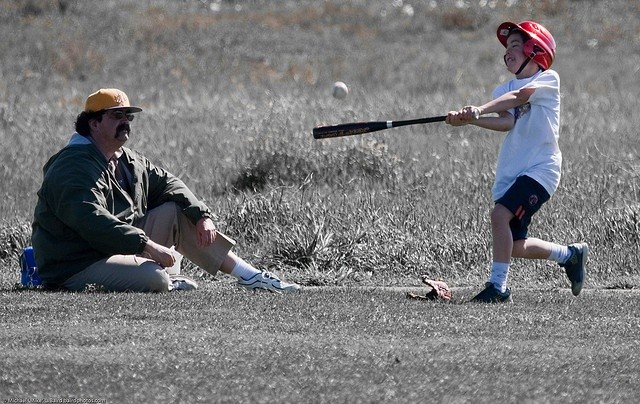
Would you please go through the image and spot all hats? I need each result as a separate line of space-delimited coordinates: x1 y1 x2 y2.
84 89 143 117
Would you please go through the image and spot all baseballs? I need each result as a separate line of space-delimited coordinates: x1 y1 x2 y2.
332 81 348 100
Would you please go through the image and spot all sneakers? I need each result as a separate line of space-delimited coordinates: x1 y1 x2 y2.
172 276 199 290
561 243 588 297
235 270 300 293
466 283 513 305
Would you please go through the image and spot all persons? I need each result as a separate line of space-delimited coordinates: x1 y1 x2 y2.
445 22 589 305
30 87 302 294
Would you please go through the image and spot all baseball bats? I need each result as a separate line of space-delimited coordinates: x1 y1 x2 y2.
313 115 448 139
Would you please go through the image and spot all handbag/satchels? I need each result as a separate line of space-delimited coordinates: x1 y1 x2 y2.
19 247 43 288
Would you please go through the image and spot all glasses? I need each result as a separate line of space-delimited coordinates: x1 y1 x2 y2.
99 112 136 121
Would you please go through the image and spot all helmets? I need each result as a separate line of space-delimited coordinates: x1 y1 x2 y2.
495 20 556 71
